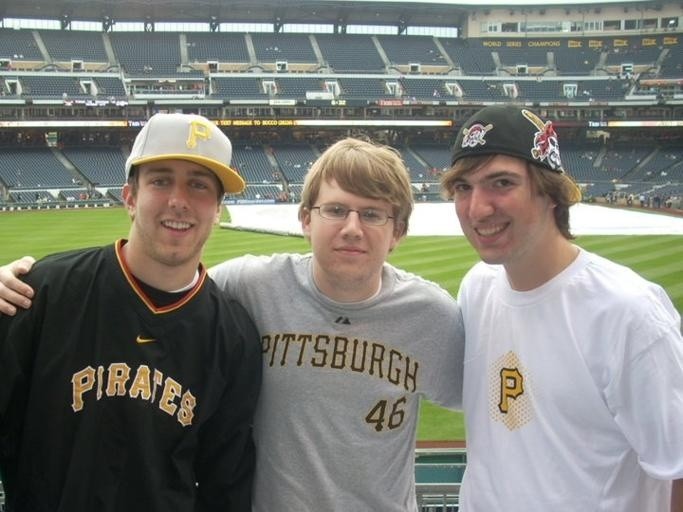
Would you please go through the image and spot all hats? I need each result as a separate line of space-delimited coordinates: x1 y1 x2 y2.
450 102 565 176
123 112 246 195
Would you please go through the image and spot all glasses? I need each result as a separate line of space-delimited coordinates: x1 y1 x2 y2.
310 201 395 228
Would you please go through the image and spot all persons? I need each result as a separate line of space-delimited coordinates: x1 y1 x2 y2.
230 158 314 203
406 163 452 201
580 149 680 209
16 177 93 202
437 102 683 511
0 112 262 512
0 134 468 511
